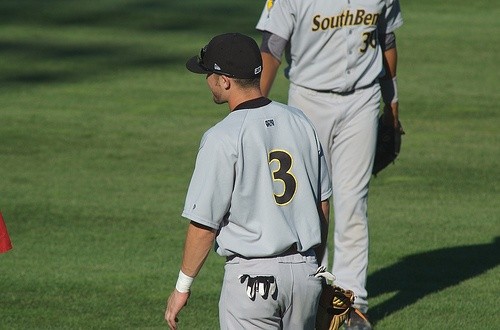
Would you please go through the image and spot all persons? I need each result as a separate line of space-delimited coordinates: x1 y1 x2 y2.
162 69 312 330
257 0 403 330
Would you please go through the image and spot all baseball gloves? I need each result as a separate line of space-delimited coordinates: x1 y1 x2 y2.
316 285 372 330
372 119 401 174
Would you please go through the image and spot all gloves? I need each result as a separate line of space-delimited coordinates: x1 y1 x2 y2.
314 266 336 280
238 274 277 299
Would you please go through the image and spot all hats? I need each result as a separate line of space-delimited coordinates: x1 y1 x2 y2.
186 33 262 79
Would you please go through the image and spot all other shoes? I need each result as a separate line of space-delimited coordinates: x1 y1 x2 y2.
343 309 371 330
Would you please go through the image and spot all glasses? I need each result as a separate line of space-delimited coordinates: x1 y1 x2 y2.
198 48 235 77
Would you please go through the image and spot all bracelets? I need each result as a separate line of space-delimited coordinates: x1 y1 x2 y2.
175 270 194 293
